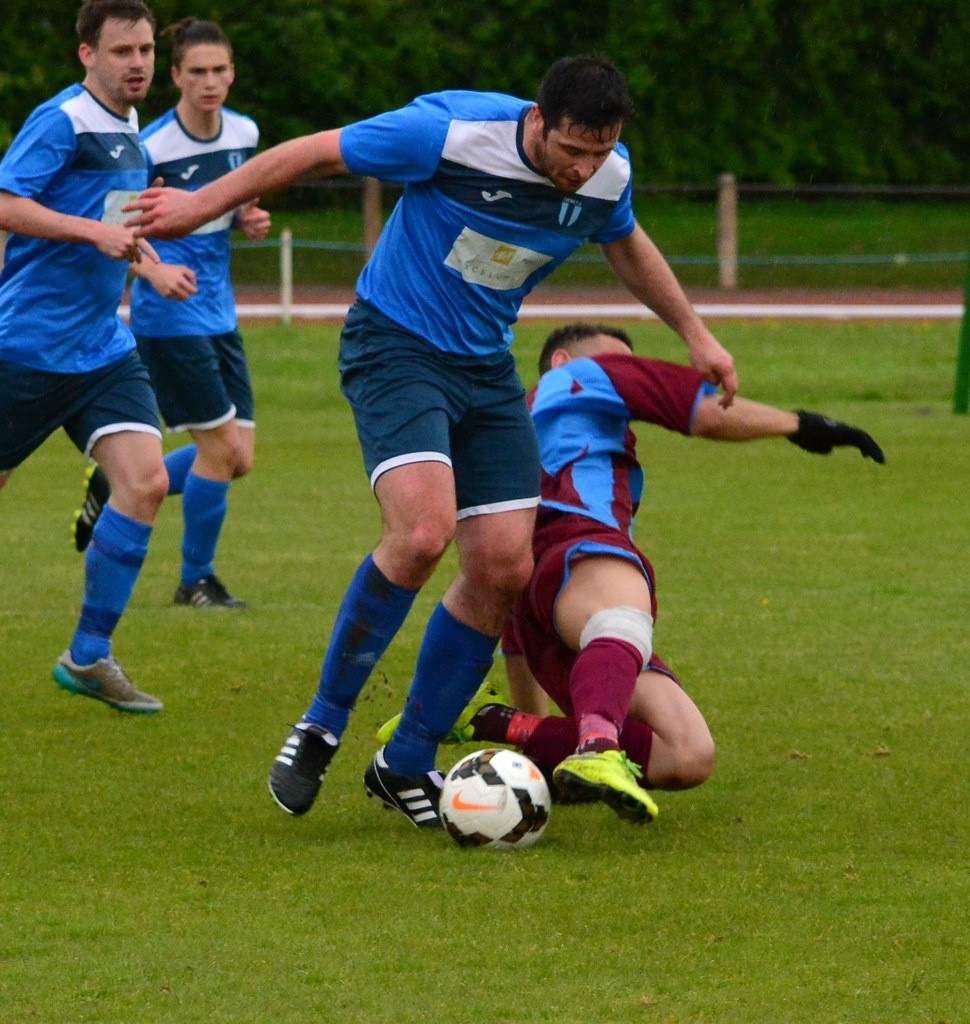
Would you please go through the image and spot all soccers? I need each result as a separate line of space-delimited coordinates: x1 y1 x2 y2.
438 748 550 854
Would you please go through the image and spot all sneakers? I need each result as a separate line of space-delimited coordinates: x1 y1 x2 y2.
553 750 659 823
53 649 165 713
267 713 340 815
362 745 448 833
174 574 247 608
70 463 112 553
377 681 509 749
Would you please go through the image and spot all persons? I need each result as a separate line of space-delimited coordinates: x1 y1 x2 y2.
119 46 744 820
377 313 891 826
64 11 277 613
0 1 170 715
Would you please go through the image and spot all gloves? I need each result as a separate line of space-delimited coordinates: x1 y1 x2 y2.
788 408 885 465
